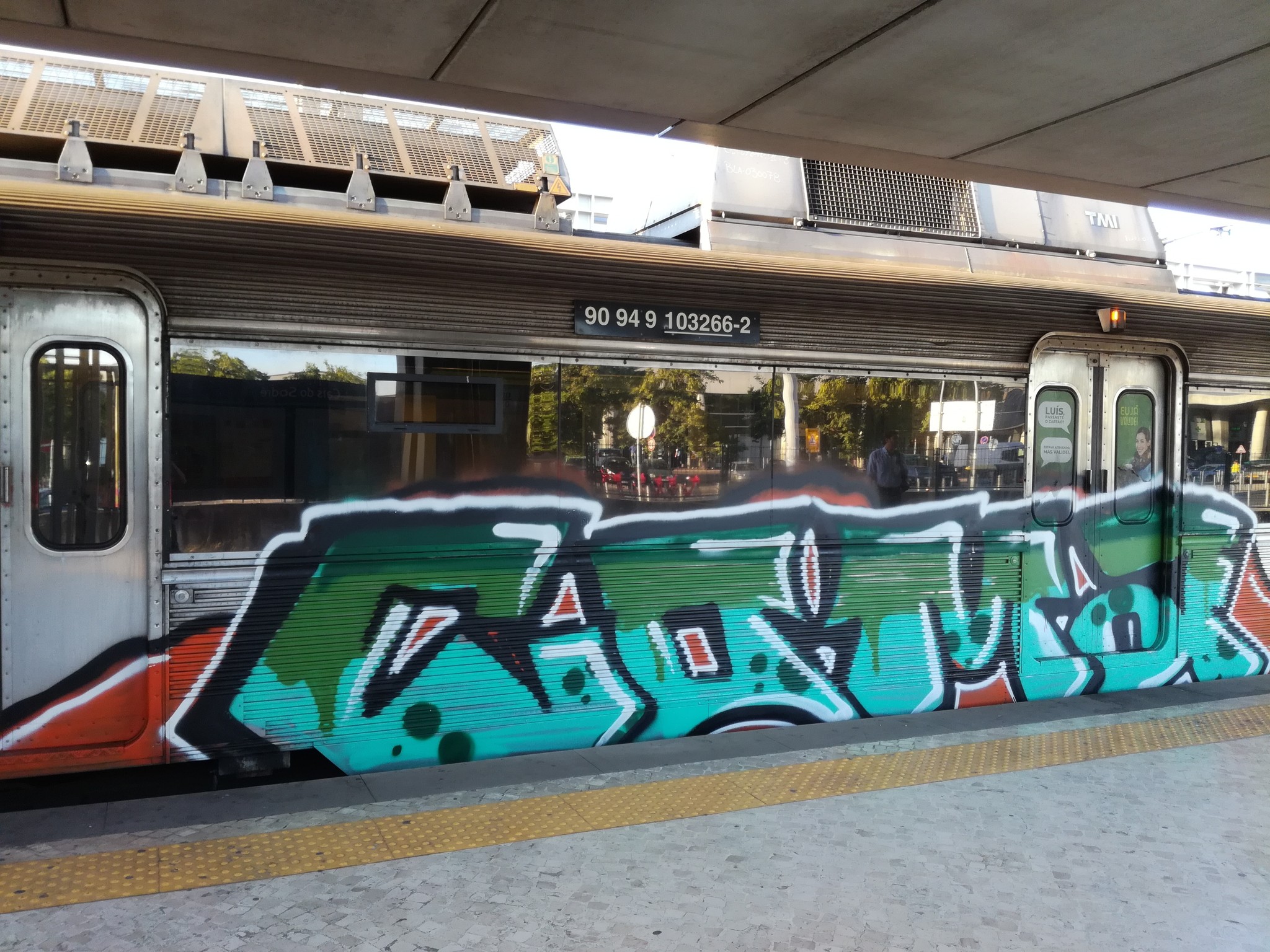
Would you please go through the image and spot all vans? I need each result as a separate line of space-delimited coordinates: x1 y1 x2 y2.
904 454 958 486
953 442 1024 485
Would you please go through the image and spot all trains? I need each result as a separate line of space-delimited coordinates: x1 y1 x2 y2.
1 42 1270 775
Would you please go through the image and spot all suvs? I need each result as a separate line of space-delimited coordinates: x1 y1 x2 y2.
593 448 621 469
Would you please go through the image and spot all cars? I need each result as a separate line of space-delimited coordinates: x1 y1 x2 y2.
1242 459 1270 484
720 460 759 486
1186 464 1226 482
598 457 637 485
640 459 675 486
563 455 590 471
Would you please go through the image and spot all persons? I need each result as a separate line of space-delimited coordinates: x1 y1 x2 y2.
629 443 636 465
866 431 907 508
663 446 684 469
691 444 704 470
